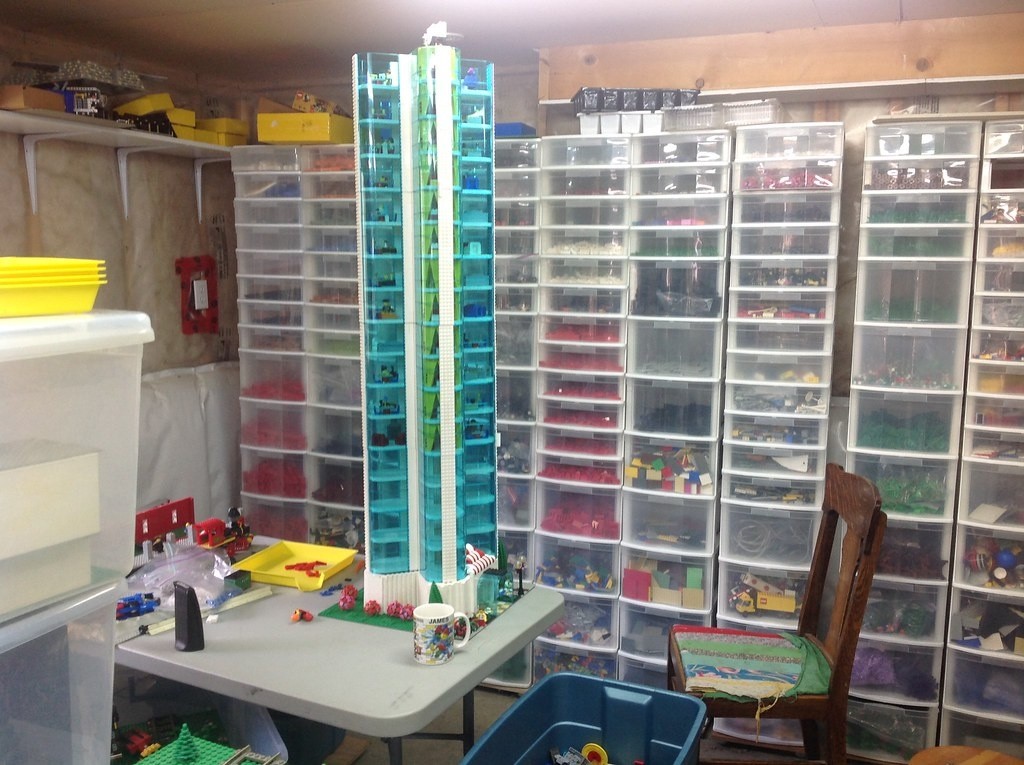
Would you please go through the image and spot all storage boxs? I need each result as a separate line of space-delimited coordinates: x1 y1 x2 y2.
1 59 1024 763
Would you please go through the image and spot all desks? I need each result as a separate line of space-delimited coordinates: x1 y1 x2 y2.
69 533 567 764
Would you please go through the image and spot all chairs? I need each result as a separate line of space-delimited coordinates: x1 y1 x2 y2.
664 464 887 764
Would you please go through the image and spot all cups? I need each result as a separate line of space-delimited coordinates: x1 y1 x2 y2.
413 602 471 667
